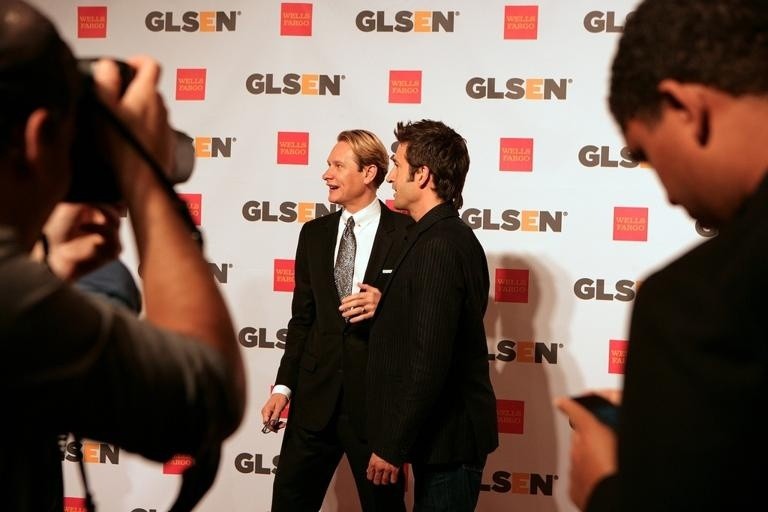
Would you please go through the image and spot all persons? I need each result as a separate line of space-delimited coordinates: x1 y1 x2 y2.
365 119 499 511
261 130 415 512
553 0 767 512
0 1 248 512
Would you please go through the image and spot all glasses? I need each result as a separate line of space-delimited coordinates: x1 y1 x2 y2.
262 417 277 433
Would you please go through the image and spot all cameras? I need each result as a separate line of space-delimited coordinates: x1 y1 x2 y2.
569 393 619 434
60 58 195 217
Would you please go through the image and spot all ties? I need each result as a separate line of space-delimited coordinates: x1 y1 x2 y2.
334 217 356 323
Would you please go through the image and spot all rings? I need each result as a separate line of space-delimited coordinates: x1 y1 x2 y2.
361 307 366 314
